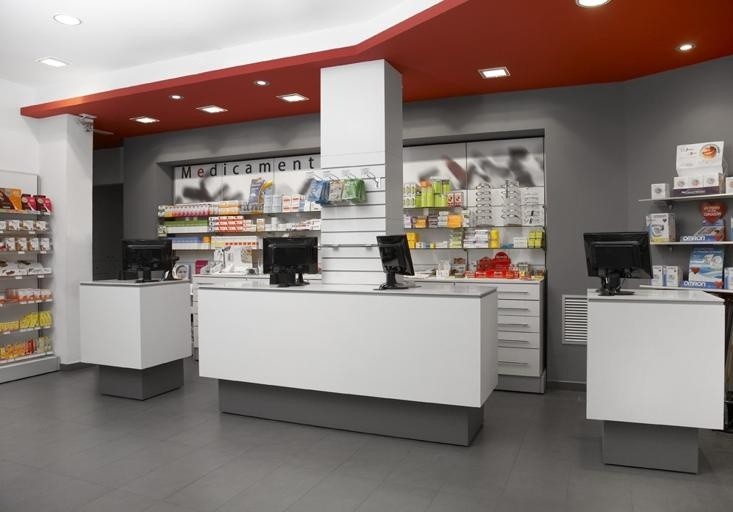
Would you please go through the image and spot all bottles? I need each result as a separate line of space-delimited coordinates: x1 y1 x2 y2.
415 176 450 207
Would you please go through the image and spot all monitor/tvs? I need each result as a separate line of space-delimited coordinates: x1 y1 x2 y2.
376 234 415 289
262 236 319 286
121 239 173 282
583 231 654 296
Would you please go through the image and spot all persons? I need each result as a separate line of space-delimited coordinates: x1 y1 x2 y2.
176 265 187 279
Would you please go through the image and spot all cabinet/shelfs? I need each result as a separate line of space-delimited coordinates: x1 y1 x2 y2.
455 283 547 395
193 276 246 361
157 205 549 251
0 170 62 383
639 193 733 294
